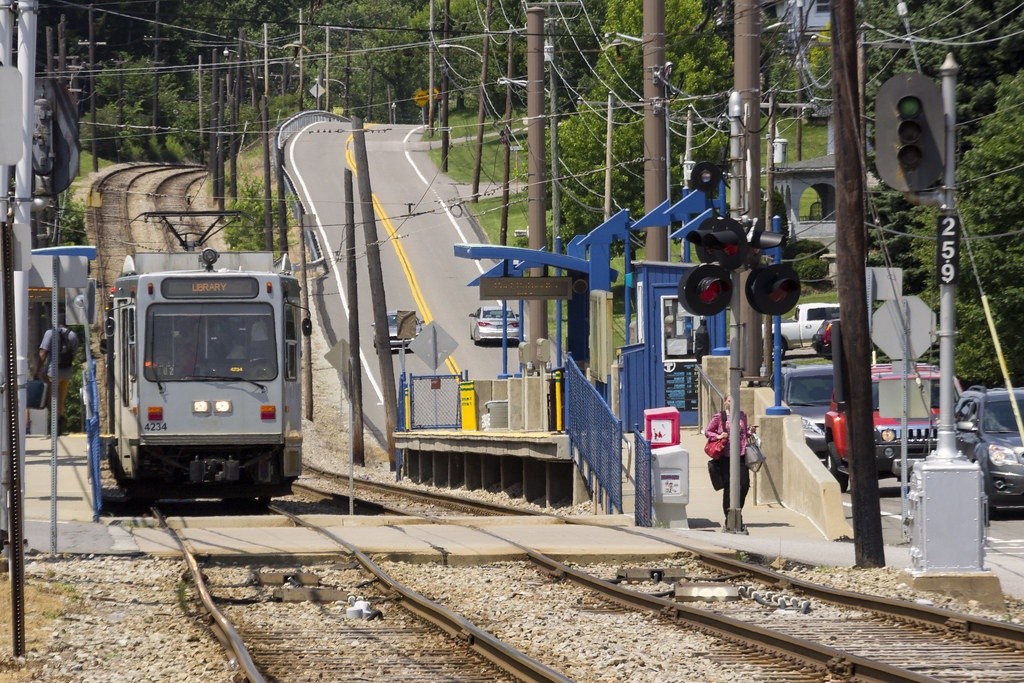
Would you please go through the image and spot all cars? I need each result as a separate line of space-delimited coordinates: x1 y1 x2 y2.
468 306 519 345
372 311 425 355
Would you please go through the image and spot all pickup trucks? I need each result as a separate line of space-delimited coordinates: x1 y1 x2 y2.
764 303 842 361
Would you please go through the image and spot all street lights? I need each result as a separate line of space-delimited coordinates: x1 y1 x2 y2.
497 77 561 260
437 43 490 205
605 30 671 263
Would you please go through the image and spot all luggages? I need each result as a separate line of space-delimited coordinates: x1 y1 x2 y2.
26 364 49 436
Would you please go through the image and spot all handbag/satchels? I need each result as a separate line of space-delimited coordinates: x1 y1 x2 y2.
708 458 730 491
704 411 728 460
744 433 765 472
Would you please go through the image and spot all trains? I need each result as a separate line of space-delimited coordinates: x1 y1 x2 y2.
100 251 312 510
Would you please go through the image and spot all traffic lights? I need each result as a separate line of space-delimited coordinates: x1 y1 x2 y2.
745 265 799 314
875 72 948 193
677 218 746 321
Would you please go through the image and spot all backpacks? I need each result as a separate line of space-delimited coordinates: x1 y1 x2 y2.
50 327 74 367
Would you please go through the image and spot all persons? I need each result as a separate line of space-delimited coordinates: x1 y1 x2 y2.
705 394 757 528
39 313 79 437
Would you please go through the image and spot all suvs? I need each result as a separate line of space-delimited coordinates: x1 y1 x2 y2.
811 319 833 359
823 362 967 491
768 361 832 454
956 387 1024 524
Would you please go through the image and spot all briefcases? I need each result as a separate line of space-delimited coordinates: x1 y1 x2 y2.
26 373 48 410
